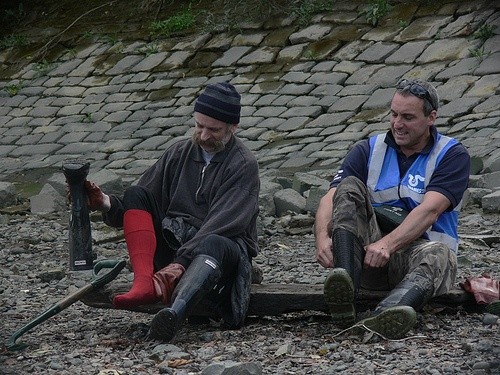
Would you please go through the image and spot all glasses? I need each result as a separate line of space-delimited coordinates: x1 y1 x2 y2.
396 78 437 111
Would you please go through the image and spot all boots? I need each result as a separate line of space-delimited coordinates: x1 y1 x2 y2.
346 279 427 341
60 158 94 271
323 227 368 330
143 255 224 342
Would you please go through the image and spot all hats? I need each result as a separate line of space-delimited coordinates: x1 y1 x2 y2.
194 82 241 124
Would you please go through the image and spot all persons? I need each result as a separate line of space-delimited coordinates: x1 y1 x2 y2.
313 79 471 343
64 80 261 343
